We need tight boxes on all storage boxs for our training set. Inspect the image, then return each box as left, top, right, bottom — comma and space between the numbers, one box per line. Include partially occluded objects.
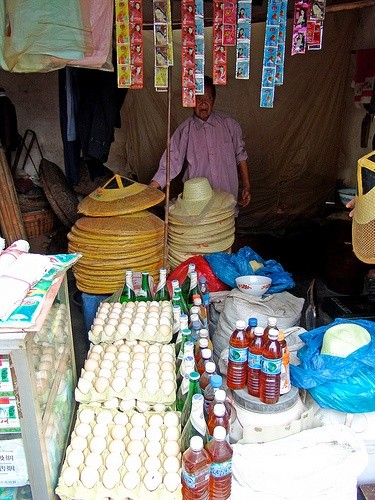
82, 293, 111, 347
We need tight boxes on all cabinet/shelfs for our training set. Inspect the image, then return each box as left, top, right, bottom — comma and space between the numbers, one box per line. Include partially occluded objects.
326, 212, 368, 296
0, 272, 78, 500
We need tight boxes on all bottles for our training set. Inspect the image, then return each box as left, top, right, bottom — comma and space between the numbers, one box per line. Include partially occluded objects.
227, 317, 291, 404
119, 263, 234, 500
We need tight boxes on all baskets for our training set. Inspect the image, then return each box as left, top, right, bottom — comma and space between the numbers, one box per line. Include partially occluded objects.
21, 209, 54, 238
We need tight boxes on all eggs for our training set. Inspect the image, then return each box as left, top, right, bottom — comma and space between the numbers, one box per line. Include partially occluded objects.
63, 301, 180, 499
33, 302, 69, 452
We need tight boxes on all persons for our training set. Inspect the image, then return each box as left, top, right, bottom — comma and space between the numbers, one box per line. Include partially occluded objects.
294, 8, 307, 52
150, 75, 252, 218
216, 2, 225, 83
117, 2, 130, 85
131, 2, 141, 84
0, 87, 23, 172
346, 150, 375, 218
184, 4, 195, 104
237, 7, 246, 76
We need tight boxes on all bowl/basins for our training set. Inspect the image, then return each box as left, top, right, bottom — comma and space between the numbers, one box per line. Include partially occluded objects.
338, 188, 359, 209
235, 275, 272, 298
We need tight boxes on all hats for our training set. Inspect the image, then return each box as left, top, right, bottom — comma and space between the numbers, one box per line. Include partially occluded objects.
310, 324, 375, 410
352, 150, 375, 264
67, 175, 235, 294
